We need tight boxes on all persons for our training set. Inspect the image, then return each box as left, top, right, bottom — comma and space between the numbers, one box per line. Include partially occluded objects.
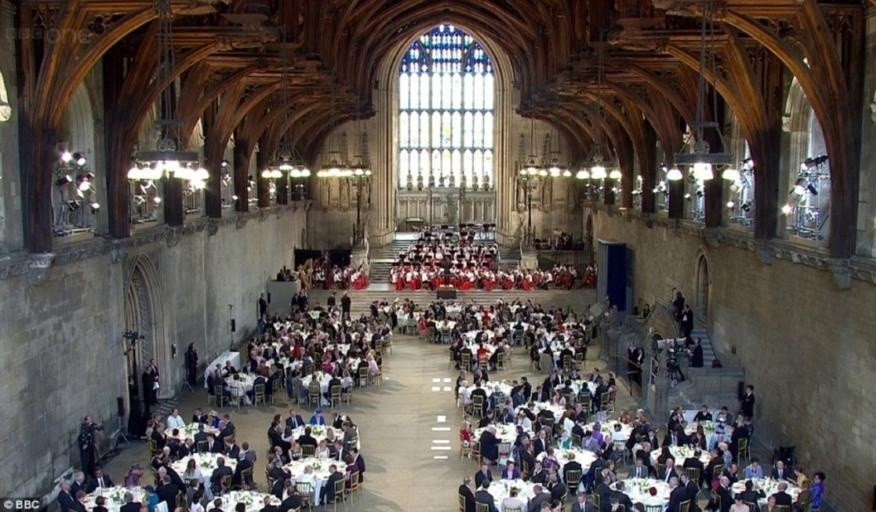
58, 234, 830, 511
277, 251, 370, 290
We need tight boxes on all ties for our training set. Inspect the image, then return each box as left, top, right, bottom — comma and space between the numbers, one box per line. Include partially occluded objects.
101, 478, 103, 488
